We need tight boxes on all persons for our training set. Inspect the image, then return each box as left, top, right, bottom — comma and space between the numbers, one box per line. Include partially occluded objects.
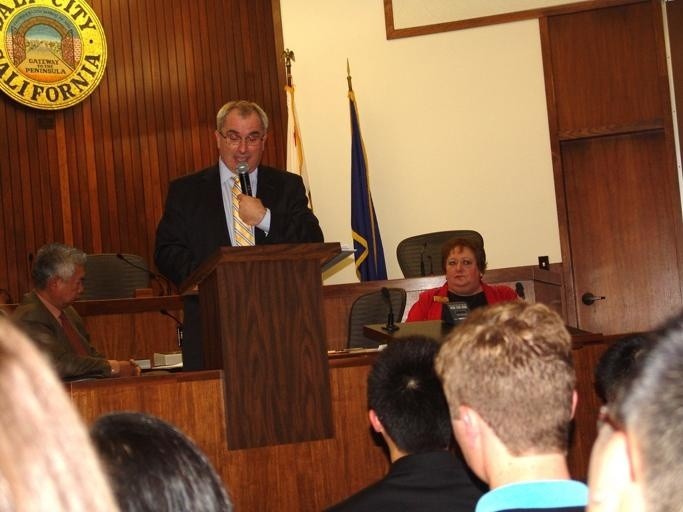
589, 312, 682, 512
323, 334, 488, 512
11, 243, 142, 381
0, 313, 121, 510
155, 100, 325, 369
434, 301, 588, 512
594, 333, 651, 405
406, 238, 520, 339
87, 410, 232, 510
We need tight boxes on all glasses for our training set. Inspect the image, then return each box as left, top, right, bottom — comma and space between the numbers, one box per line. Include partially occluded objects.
218, 131, 264, 145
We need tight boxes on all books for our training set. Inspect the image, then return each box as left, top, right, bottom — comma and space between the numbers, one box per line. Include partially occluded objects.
134, 351, 183, 370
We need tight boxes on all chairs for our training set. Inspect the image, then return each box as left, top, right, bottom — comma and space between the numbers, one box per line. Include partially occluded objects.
391, 230, 488, 277
342, 288, 406, 350
74, 253, 153, 298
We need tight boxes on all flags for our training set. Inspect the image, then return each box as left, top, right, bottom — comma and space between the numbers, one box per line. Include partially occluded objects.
285, 84, 313, 211
351, 89, 388, 281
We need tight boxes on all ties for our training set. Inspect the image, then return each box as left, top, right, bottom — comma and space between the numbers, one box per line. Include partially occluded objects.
232, 175, 253, 247
59, 311, 88, 357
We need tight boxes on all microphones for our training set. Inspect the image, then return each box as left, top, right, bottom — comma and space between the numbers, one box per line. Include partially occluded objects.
427, 256, 434, 276
420, 241, 427, 275
237, 163, 253, 196
160, 307, 182, 328
116, 252, 169, 284
382, 286, 398, 333
